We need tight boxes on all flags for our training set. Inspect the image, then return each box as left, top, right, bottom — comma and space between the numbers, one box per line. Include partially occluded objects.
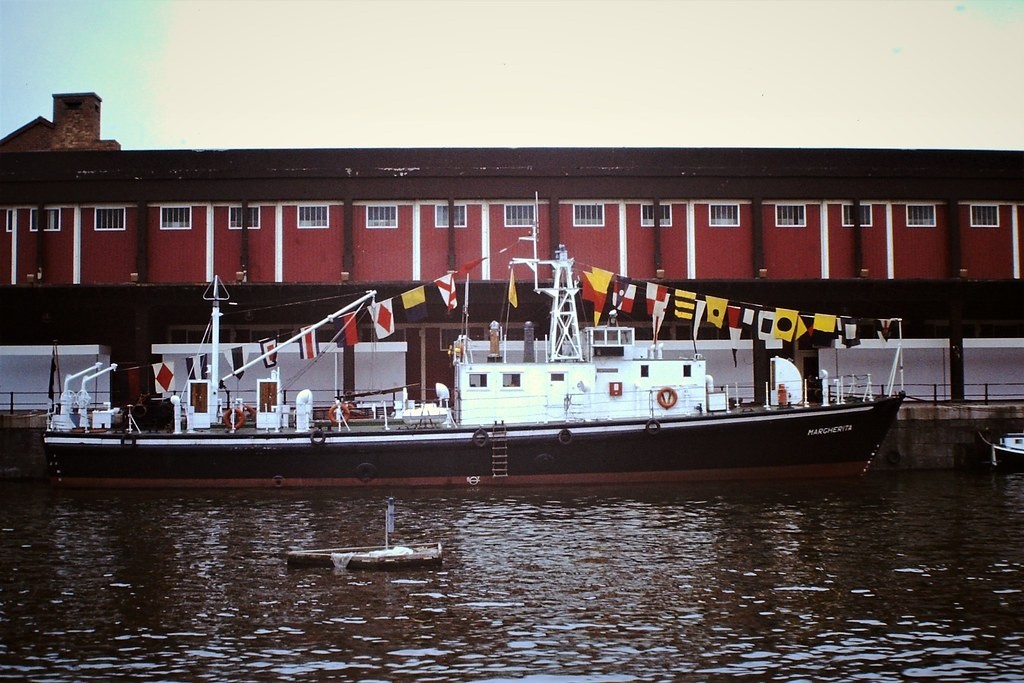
754, 306, 813, 350
675, 289, 696, 320
258, 335, 278, 369
185, 353, 208, 380
840, 316, 861, 346
812, 312, 836, 348
48, 347, 57, 409
298, 324, 320, 360
582, 270, 610, 305
737, 301, 763, 331
366, 297, 395, 340
646, 281, 669, 315
222, 343, 250, 381
333, 309, 359, 348
433, 273, 458, 309
705, 295, 729, 329
151, 360, 176, 394
401, 285, 426, 309
509, 267, 519, 309
612, 279, 637, 314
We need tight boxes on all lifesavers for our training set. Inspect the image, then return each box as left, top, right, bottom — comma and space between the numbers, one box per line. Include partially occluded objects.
355, 463, 377, 482
658, 387, 678, 407
557, 428, 573, 445
132, 404, 147, 418
329, 404, 351, 425
310, 429, 326, 445
646, 419, 661, 434
223, 408, 246, 429
473, 429, 489, 446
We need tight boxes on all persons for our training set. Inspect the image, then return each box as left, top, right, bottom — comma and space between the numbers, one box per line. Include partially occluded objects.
607, 309, 619, 327
334, 396, 341, 405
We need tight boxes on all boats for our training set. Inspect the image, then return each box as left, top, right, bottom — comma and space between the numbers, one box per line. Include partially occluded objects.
972, 428, 1024, 471
41, 189, 909, 489
